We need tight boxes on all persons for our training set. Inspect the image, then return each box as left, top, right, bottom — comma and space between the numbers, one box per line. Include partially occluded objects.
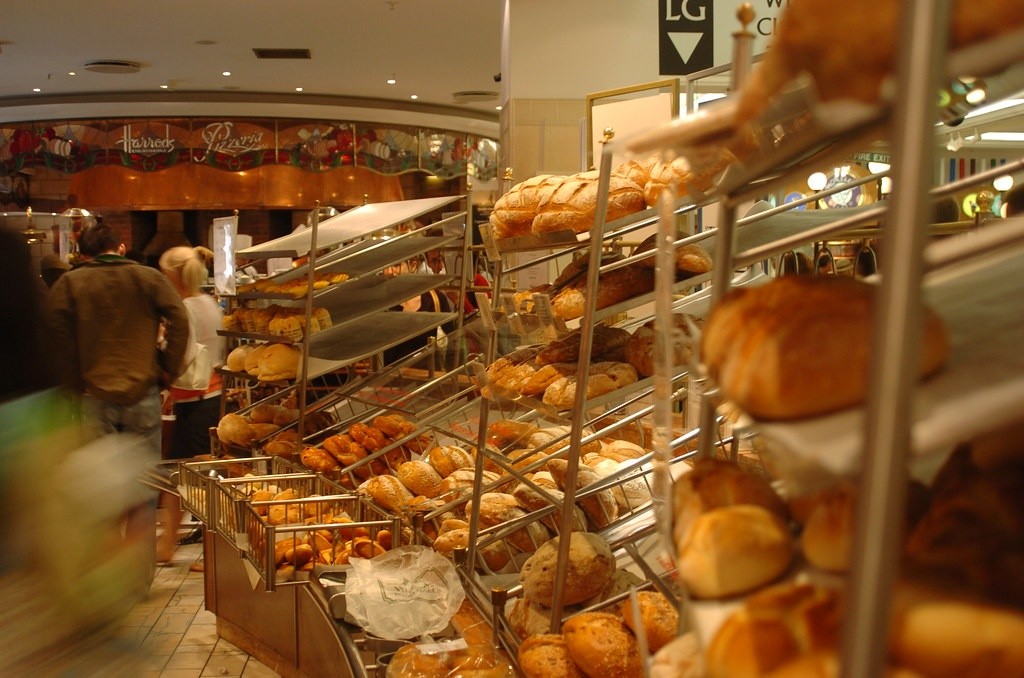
49, 224, 224, 571
384, 219, 493, 367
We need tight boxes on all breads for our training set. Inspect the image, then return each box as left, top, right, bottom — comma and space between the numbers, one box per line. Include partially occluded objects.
216, 271, 340, 458
190, 410, 439, 584
390, 0, 1024, 678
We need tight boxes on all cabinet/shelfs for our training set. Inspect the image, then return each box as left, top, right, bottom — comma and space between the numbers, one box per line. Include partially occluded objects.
142, 1, 1024, 677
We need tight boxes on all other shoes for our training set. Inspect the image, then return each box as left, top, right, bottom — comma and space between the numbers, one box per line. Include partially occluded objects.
155, 559, 173, 568
177, 528, 203, 545
